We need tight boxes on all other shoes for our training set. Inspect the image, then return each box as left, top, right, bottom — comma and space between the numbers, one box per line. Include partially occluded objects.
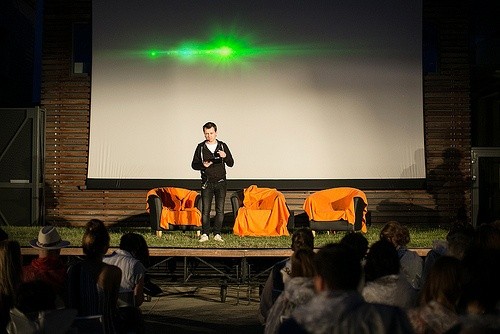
198, 234, 208, 244
213, 234, 224, 242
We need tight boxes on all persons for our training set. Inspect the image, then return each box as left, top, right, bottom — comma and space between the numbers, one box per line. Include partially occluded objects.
258, 228, 314, 323
103, 233, 150, 334
338, 220, 500, 333
263, 246, 316, 334
192, 122, 234, 243
0, 225, 76, 333
274, 242, 413, 334
60, 219, 122, 334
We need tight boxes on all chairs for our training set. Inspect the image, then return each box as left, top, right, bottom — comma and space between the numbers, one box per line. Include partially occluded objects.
230, 185, 295, 236
118, 290, 139, 333
302, 186, 369, 234
146, 187, 204, 236
74, 314, 105, 334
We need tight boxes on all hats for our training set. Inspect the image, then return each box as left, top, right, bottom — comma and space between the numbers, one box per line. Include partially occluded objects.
29, 227, 70, 250
